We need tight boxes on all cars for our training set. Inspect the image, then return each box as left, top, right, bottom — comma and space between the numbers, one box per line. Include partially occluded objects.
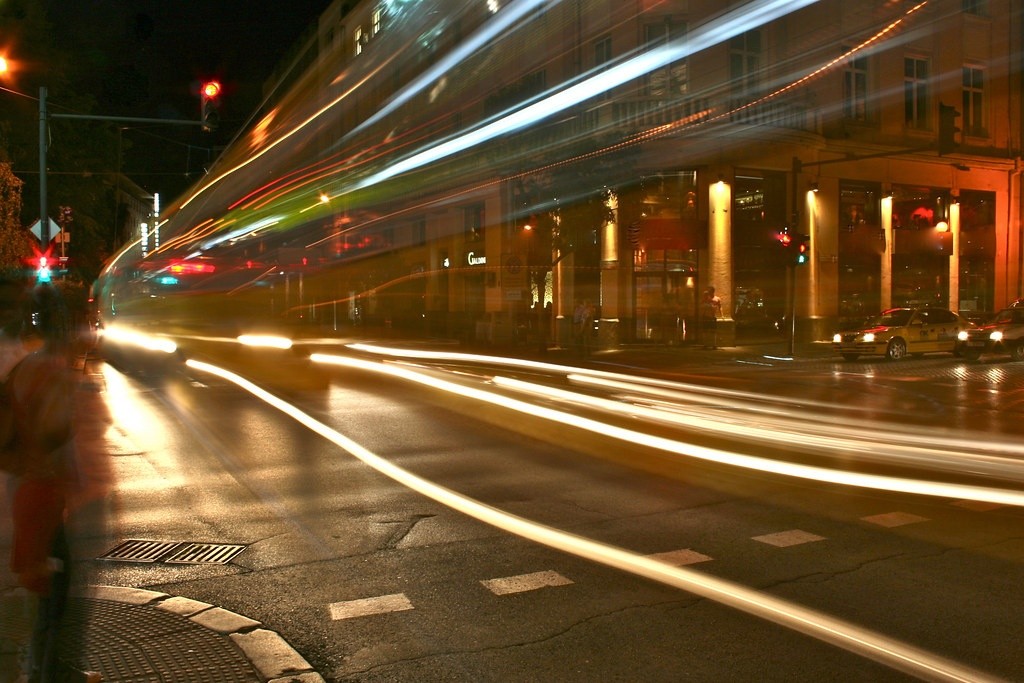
831, 303, 978, 361
955, 298, 1024, 361
733, 291, 978, 330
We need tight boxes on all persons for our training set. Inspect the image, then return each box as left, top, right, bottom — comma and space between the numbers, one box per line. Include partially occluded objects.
700, 291, 717, 319
573, 298, 590, 353
0, 287, 115, 683
702, 285, 725, 349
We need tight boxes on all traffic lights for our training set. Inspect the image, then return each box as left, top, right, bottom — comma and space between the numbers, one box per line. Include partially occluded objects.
202, 78, 220, 133
932, 217, 951, 258
936, 104, 960, 157
792, 233, 811, 267
34, 253, 55, 289
780, 222, 790, 250
